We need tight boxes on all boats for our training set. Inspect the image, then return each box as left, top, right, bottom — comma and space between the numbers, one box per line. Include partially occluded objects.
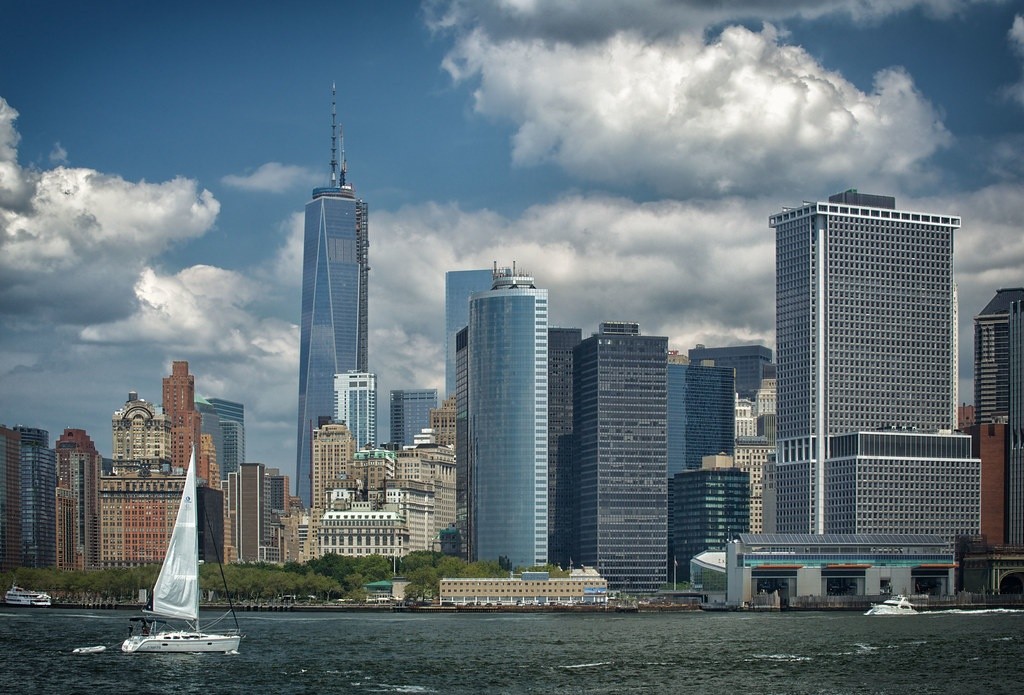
72, 644, 107, 655
863, 592, 920, 616
5, 586, 53, 608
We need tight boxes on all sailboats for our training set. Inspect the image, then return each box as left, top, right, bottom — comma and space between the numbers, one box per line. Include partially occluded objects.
117, 440, 242, 654
